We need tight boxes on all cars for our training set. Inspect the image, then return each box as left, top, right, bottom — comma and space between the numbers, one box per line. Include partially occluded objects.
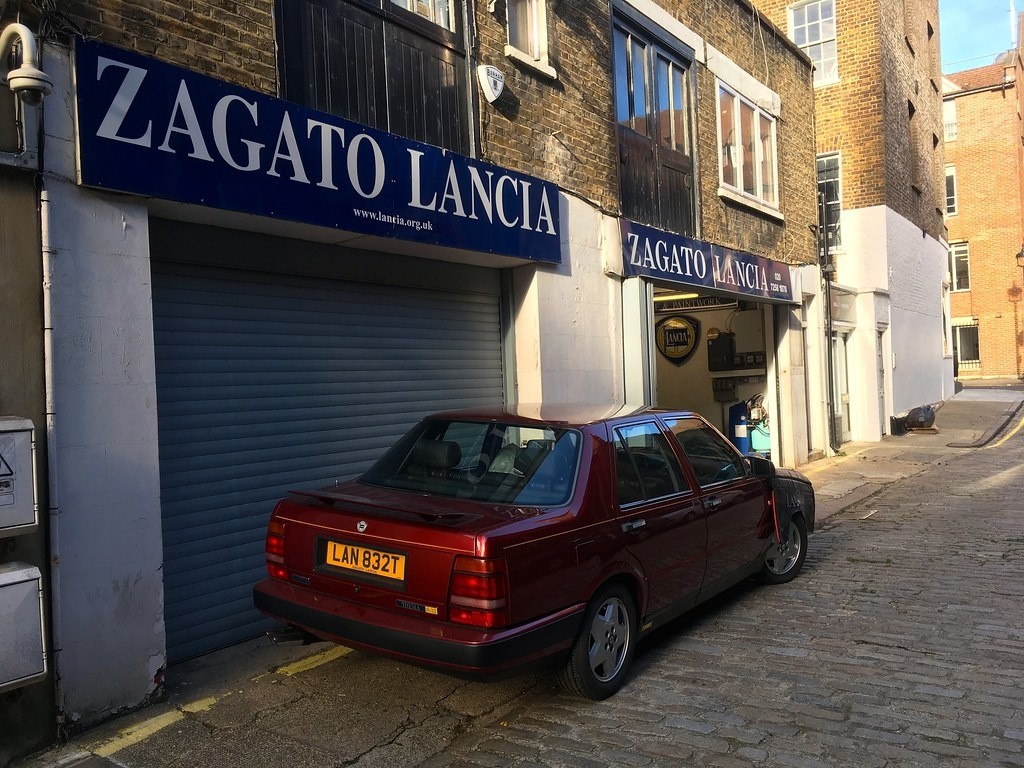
254, 400, 816, 704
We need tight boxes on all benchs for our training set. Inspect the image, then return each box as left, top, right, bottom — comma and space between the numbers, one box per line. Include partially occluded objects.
411, 440, 567, 504
